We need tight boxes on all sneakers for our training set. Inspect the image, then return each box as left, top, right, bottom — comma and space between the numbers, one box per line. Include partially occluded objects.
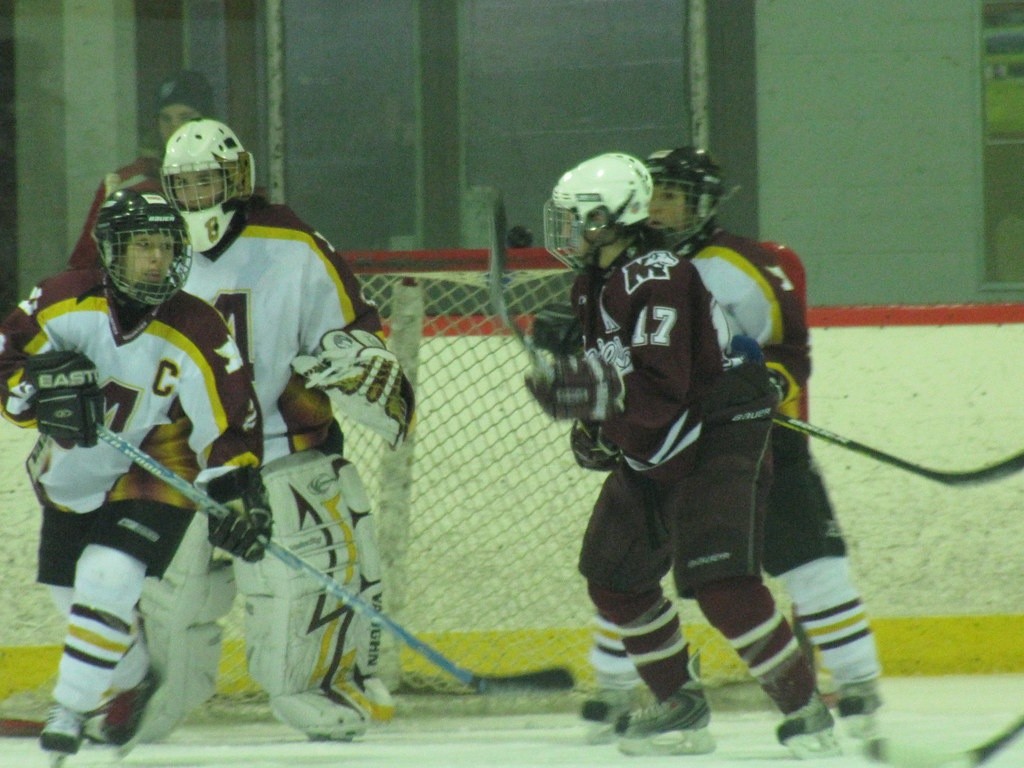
838, 680, 885, 753
97, 644, 176, 760
582, 673, 651, 745
777, 689, 842, 759
616, 650, 718, 755
41, 703, 88, 768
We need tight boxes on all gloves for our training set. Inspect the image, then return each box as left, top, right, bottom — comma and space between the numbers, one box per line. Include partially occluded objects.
525, 349, 625, 418
570, 420, 627, 470
531, 304, 575, 350
207, 465, 275, 566
769, 368, 788, 403
28, 349, 103, 448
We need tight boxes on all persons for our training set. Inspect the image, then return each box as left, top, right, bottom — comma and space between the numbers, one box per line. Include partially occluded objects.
0, 186, 274, 754
70, 72, 417, 741
521, 152, 839, 760
578, 147, 886, 759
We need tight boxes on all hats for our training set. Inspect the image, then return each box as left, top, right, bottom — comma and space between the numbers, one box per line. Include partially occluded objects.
158, 70, 213, 115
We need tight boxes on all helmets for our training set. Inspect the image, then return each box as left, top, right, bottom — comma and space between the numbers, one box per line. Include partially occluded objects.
646, 145, 729, 251
541, 153, 653, 271
162, 118, 255, 252
91, 187, 188, 304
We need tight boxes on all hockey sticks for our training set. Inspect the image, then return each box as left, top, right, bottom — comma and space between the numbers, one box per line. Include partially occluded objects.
864, 715, 1024, 767
94, 420, 578, 696
485, 192, 544, 370
765, 407, 1020, 489
